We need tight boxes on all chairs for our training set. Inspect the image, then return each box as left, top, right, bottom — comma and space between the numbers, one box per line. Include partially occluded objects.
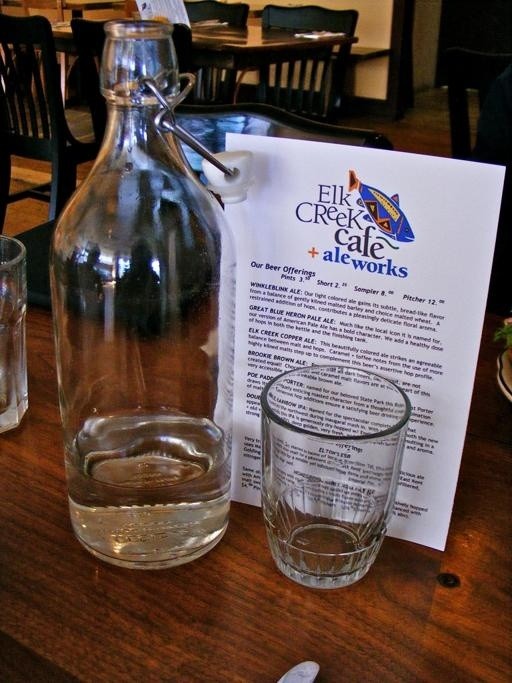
0, 13, 105, 222
260, 4, 359, 121
184, 0, 251, 106
442, 46, 510, 231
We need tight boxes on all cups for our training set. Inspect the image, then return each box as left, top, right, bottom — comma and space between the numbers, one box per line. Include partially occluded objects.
260, 362, 412, 592
0, 235, 30, 437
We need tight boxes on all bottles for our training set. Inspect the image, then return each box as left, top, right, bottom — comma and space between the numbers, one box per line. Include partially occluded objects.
48, 21, 238, 575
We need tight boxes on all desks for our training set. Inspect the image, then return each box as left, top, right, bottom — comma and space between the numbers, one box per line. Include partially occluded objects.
0, 238, 511, 683
0, 1, 361, 124
